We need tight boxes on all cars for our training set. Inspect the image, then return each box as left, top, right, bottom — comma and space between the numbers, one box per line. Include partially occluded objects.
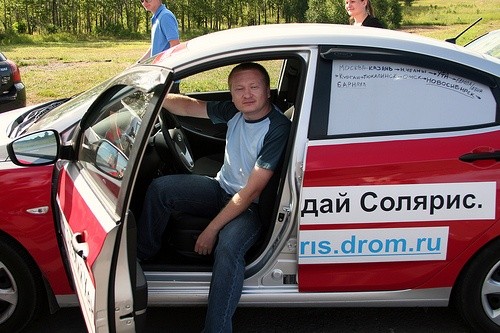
0, 23, 499, 333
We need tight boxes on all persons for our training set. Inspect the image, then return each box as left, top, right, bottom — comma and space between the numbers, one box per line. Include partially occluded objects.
345, 0, 384, 28
138, 1, 180, 94
137, 62, 292, 333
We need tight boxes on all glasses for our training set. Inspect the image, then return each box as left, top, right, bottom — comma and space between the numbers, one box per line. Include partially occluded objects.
140, 0, 151, 3
